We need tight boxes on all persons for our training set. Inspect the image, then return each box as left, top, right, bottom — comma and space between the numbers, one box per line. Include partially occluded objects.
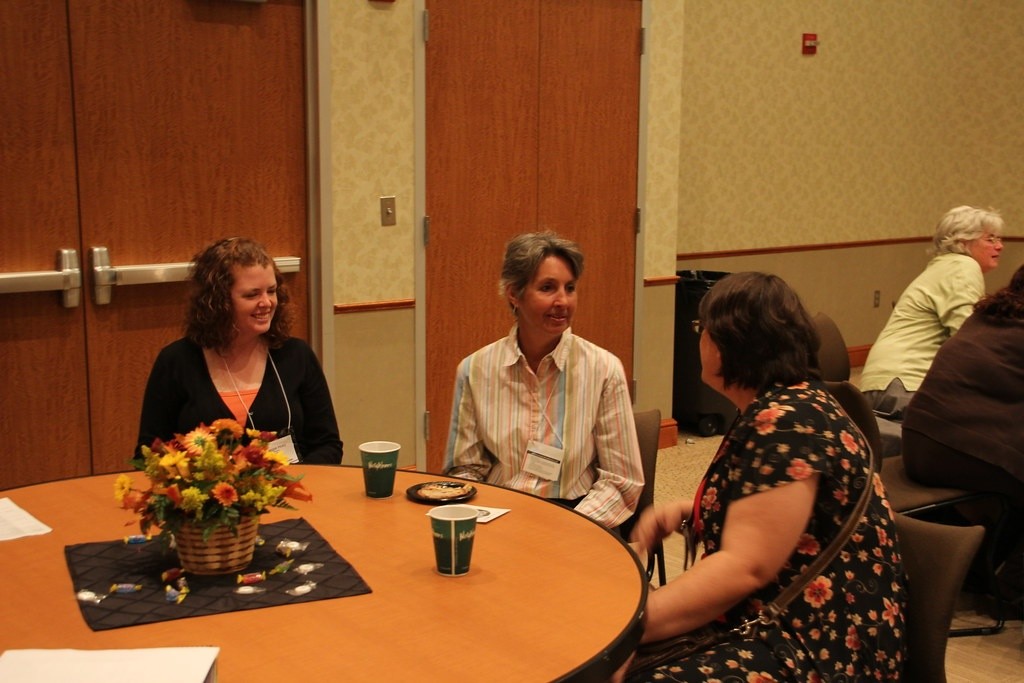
610, 270, 911, 683
131, 233, 343, 467
899, 262, 1024, 623
857, 206, 1005, 426
441, 229, 644, 540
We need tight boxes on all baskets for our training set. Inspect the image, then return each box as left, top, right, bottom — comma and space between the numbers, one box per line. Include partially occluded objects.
170, 514, 258, 575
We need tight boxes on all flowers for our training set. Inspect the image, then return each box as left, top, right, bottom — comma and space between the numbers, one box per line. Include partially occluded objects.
115, 418, 314, 556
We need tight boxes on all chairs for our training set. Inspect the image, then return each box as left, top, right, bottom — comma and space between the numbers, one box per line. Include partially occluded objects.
812, 310, 1009, 636
614, 411, 666, 587
892, 510, 986, 683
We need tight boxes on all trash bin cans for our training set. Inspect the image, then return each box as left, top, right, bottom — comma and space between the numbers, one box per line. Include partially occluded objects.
672, 270, 740, 436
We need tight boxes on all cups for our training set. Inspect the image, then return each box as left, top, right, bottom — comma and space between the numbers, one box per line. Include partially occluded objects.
358, 440, 401, 499
425, 504, 479, 577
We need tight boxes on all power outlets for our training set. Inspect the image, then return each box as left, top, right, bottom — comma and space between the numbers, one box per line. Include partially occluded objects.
874, 290, 880, 307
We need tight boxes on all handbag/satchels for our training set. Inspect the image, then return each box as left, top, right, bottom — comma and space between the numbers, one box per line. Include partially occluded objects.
623, 624, 717, 677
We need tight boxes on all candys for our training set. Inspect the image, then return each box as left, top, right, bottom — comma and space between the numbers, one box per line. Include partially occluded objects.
76, 535, 316, 604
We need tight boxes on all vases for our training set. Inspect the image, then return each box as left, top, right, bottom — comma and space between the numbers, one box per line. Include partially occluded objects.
173, 516, 259, 575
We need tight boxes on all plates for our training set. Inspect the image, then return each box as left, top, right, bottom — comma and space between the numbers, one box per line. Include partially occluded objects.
406, 481, 477, 504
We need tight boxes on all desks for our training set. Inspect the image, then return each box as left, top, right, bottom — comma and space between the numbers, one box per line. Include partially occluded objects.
0, 463, 649, 683
